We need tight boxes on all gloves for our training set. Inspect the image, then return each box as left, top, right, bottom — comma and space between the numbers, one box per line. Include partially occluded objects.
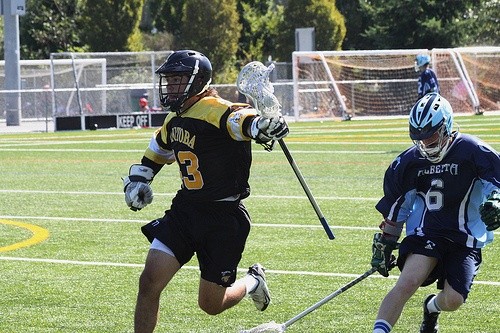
479, 193, 500, 232
370, 231, 398, 277
251, 115, 289, 144
122, 164, 156, 211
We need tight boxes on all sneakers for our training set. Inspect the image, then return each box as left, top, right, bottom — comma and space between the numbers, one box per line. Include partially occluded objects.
248, 262, 270, 312
420, 293, 441, 333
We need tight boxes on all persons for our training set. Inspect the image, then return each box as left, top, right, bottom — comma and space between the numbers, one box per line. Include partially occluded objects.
370, 91, 500, 333
139, 93, 150, 111
121, 49, 289, 333
414, 54, 440, 99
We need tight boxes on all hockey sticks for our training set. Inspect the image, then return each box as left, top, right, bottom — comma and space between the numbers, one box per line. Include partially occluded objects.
240, 255, 395, 333
235, 60, 335, 240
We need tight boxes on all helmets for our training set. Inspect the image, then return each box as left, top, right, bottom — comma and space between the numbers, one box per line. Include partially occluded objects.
155, 49, 213, 112
415, 54, 429, 71
408, 92, 453, 162
